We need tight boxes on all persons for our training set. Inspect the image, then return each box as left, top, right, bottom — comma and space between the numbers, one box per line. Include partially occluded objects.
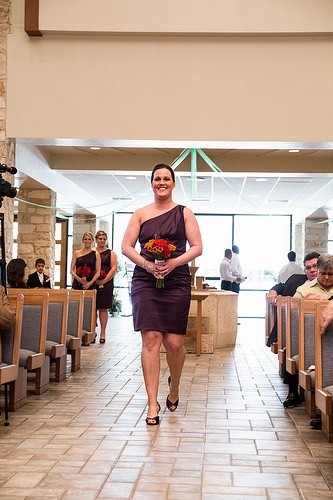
27, 258, 51, 289
70, 232, 101, 346
0, 285, 16, 327
278, 251, 304, 283
293, 254, 333, 427
320, 295, 333, 334
121, 164, 203, 424
7, 258, 28, 289
90, 231, 117, 343
220, 245, 242, 325
266, 252, 321, 407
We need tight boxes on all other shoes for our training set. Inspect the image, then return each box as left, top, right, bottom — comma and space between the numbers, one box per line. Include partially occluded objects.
310, 418, 323, 427
282, 390, 301, 407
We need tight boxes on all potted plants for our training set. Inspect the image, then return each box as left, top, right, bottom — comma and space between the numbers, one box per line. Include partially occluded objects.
108, 289, 122, 317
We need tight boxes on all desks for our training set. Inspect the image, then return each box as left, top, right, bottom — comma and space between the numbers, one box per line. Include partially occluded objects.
185, 295, 209, 358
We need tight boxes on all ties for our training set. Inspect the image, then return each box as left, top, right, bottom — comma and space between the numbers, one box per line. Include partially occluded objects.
38, 273, 44, 283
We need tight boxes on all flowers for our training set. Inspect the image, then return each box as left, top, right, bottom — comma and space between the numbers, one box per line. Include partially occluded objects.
144, 240, 176, 288
94, 270, 107, 288
76, 266, 91, 282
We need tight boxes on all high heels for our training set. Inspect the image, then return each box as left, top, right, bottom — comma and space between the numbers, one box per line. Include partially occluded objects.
145, 401, 161, 425
165, 376, 180, 412
99, 334, 106, 344
91, 334, 97, 344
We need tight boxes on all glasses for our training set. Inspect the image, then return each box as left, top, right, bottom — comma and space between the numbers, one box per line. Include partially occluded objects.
319, 271, 333, 276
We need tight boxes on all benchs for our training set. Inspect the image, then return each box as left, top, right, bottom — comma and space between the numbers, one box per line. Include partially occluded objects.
265, 293, 333, 443
0, 288, 97, 412
